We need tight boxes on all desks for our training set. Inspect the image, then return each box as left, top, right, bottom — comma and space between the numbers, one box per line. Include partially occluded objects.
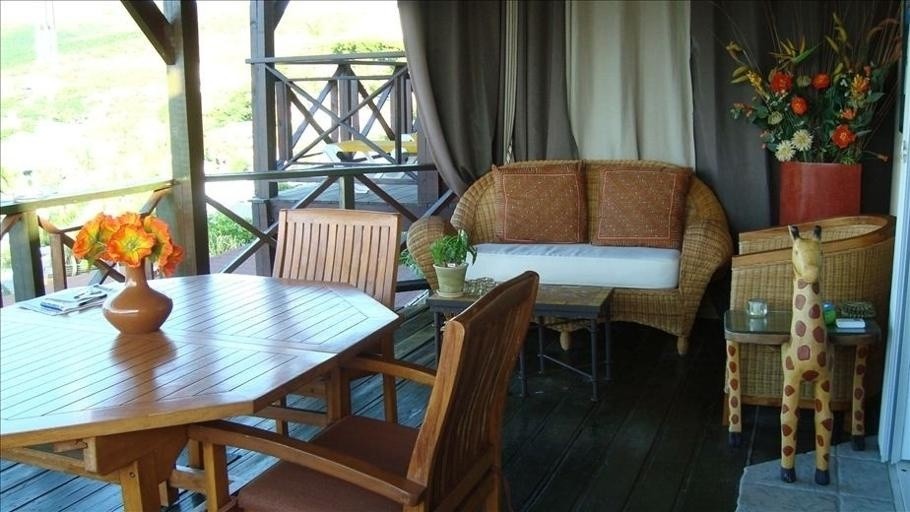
721, 224, 880, 450
3, 270, 402, 512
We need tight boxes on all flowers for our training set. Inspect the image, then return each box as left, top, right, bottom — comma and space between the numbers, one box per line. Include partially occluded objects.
725, 0, 910, 161
69, 210, 187, 277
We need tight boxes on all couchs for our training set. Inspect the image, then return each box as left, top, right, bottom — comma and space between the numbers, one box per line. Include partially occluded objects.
407, 158, 734, 360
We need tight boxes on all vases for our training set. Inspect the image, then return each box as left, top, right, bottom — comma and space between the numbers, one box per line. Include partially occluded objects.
101, 259, 177, 338
779, 161, 861, 227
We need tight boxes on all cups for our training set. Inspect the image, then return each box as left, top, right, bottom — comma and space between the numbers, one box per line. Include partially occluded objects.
746, 297, 766, 317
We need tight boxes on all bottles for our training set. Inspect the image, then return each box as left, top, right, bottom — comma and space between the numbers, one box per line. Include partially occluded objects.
822, 303, 836, 324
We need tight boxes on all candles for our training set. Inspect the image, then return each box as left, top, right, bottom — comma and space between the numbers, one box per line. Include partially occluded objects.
428, 227, 480, 298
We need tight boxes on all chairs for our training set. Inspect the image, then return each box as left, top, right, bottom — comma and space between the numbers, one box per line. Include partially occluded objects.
185, 268, 538, 512
325, 133, 419, 193
255, 207, 404, 443
722, 214, 897, 426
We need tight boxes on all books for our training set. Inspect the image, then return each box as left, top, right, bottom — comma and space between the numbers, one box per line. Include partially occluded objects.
20, 283, 113, 316
